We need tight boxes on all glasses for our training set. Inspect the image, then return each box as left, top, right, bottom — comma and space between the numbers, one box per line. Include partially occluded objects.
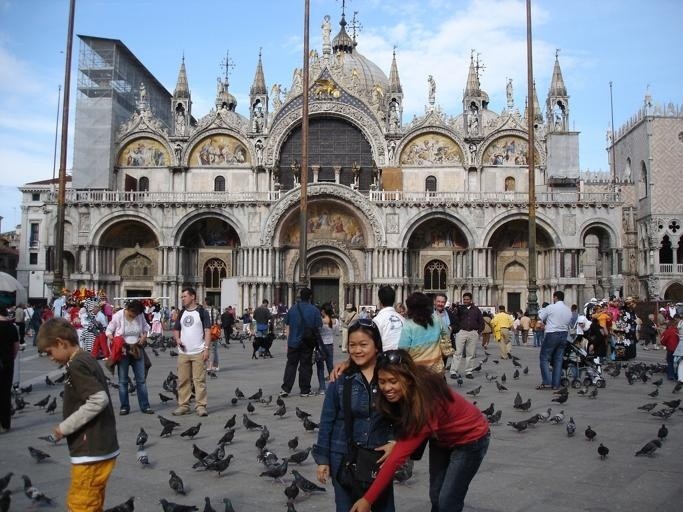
376, 351, 407, 365
348, 319, 377, 335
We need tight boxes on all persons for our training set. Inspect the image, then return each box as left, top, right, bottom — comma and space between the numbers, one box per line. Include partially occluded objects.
1, 287, 212, 435
205, 298, 683, 389
328, 350, 490, 512
279, 287, 323, 398
216, 78, 224, 98
505, 79, 513, 102
35, 318, 120, 511
146, 144, 166, 168
198, 137, 245, 167
387, 107, 398, 134
537, 290, 572, 389
125, 142, 146, 167
375, 286, 485, 379
320, 15, 332, 53
427, 75, 436, 97
139, 82, 146, 102
311, 318, 406, 511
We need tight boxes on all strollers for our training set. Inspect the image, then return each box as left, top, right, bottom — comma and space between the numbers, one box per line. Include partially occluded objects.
560, 334, 605, 390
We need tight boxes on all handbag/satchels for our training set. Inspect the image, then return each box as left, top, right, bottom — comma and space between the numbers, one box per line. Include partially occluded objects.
439, 335, 455, 357
337, 448, 385, 494
302, 328, 317, 346
125, 344, 142, 361
315, 343, 327, 365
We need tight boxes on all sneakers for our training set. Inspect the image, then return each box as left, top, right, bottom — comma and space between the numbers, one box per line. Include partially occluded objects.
173, 404, 191, 416
196, 409, 209, 417
144, 408, 155, 415
302, 390, 317, 397
119, 408, 129, 416
279, 389, 289, 397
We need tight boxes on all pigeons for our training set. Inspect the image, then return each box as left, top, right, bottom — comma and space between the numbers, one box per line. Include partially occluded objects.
598, 443, 609, 460
218, 332, 255, 349
37, 431, 61, 445
466, 350, 683, 441
145, 332, 179, 358
0, 490, 12, 512
21, 474, 52, 508
394, 466, 413, 485
9, 372, 66, 418
106, 372, 326, 512
28, 446, 51, 463
0, 472, 14, 494
635, 440, 662, 458
457, 378, 463, 387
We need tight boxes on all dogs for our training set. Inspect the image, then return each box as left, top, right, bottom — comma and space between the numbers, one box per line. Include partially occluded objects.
250, 333, 277, 359
